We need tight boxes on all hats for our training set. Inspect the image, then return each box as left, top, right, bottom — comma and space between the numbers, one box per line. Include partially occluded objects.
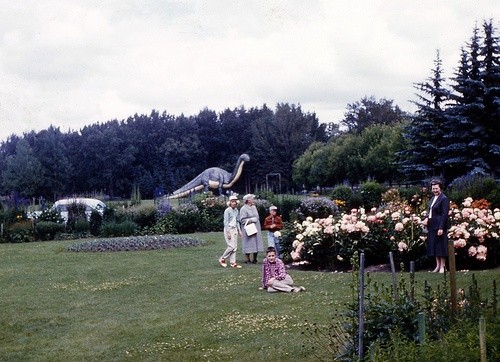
243, 193, 256, 200
227, 196, 240, 206
269, 206, 278, 210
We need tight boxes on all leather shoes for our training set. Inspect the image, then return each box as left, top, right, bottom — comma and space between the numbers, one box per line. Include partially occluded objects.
245, 259, 258, 265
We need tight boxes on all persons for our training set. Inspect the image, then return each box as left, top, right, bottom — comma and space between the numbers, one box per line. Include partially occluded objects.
240, 194, 264, 264
264, 205, 284, 258
424, 179, 449, 273
219, 196, 244, 269
258, 247, 305, 292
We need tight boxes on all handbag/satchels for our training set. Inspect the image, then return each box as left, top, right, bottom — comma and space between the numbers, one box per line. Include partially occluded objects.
243, 219, 258, 237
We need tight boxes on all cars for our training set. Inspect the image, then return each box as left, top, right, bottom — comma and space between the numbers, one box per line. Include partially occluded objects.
27, 198, 107, 225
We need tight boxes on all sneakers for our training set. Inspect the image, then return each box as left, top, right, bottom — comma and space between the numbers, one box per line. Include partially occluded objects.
292, 286, 306, 292
230, 262, 241, 269
218, 258, 226, 268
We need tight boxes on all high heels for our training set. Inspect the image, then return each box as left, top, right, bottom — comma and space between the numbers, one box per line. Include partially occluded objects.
433, 265, 445, 274
